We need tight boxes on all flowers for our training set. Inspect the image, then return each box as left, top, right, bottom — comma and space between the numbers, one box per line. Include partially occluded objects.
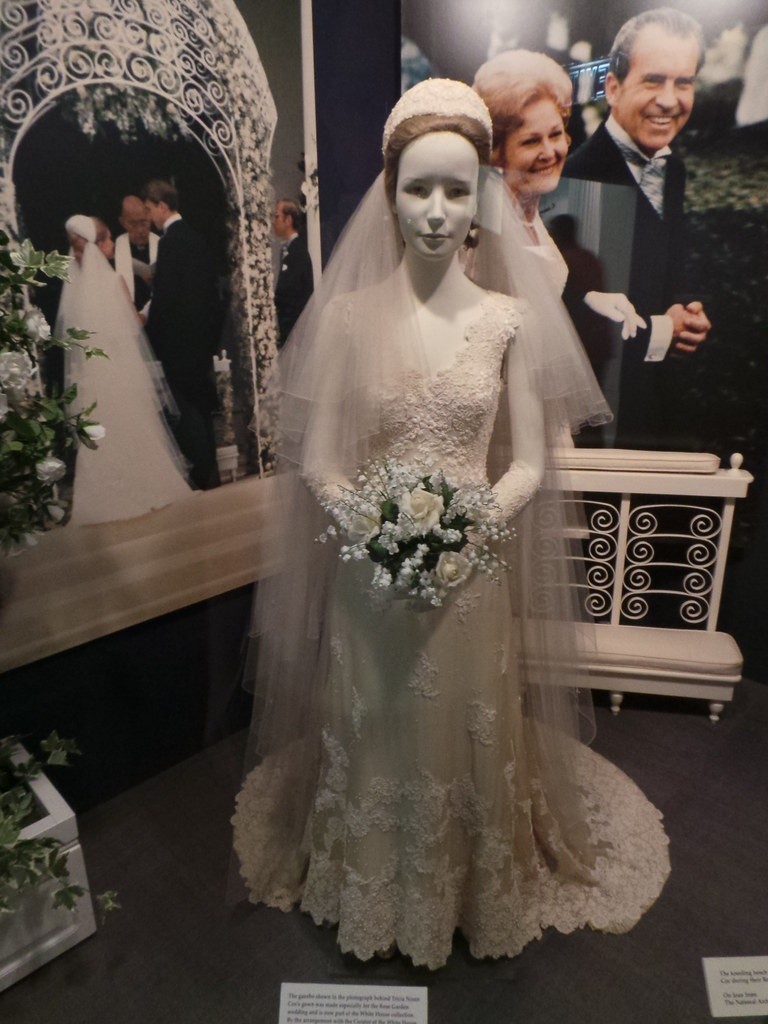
316, 457, 515, 618
0, 235, 107, 534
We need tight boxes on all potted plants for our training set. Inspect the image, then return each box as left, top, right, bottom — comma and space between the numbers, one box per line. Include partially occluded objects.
0, 739, 98, 991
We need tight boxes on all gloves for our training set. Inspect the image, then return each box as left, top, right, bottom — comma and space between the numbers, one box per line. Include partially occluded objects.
584, 288, 647, 340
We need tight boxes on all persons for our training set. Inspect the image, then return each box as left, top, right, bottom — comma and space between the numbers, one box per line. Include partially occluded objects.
141, 180, 219, 491
272, 200, 314, 344
233, 78, 668, 967
468, 49, 648, 341
107, 195, 159, 326
540, 9, 711, 448
58, 215, 195, 528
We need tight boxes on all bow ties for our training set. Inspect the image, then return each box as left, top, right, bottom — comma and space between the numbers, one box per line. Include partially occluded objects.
614, 137, 666, 184
